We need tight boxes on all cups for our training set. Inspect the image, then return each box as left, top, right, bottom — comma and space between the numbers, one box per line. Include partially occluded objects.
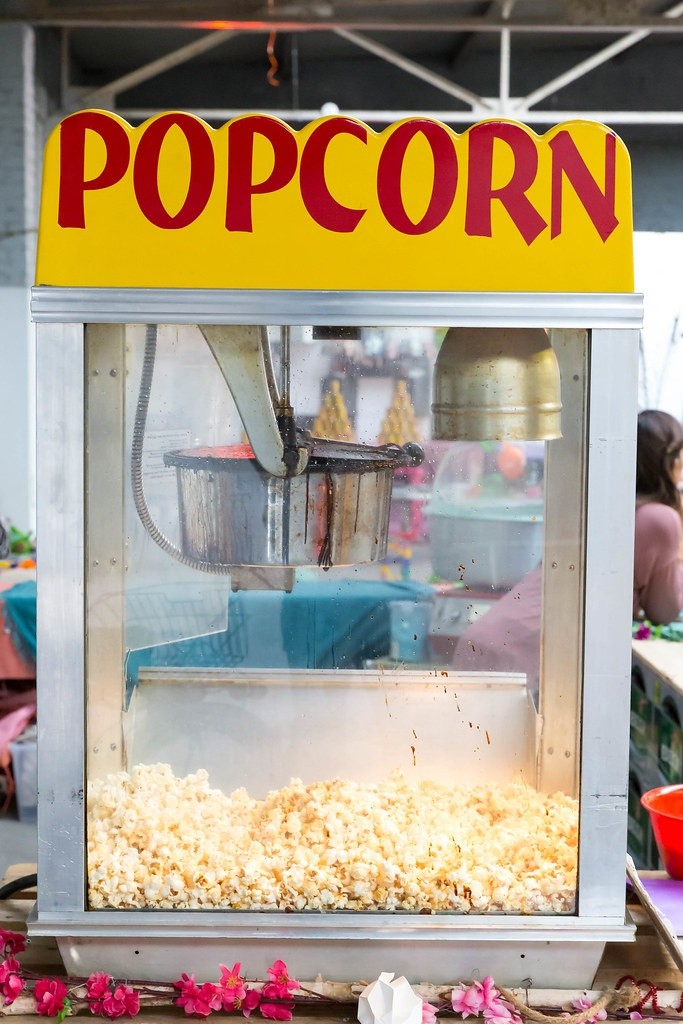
388, 600, 429, 662
6, 741, 38, 823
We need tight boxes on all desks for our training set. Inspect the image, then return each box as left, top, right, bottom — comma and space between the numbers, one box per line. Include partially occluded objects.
0, 568, 437, 681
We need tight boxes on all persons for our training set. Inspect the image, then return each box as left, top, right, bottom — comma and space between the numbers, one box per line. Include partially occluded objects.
453, 411, 683, 711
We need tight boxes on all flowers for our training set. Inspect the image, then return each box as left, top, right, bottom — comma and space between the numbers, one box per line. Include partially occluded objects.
0, 928, 683, 1024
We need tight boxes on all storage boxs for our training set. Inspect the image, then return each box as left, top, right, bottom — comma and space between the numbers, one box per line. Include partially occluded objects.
420, 440, 546, 589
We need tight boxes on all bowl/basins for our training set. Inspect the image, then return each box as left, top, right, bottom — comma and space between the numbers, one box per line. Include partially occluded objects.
421, 506, 544, 588
640, 784, 683, 879
